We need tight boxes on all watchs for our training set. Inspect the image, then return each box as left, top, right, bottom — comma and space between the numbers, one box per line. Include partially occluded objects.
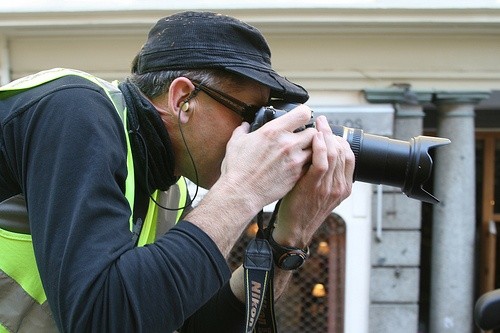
260, 228, 311, 271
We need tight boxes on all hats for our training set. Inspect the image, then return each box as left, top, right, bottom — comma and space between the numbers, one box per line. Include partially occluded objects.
131, 10, 309, 106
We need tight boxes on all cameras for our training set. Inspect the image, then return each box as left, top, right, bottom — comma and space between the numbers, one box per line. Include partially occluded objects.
248, 98, 451, 206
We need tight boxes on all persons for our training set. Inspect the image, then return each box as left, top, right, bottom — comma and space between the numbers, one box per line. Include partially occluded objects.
0, 11, 357, 333
